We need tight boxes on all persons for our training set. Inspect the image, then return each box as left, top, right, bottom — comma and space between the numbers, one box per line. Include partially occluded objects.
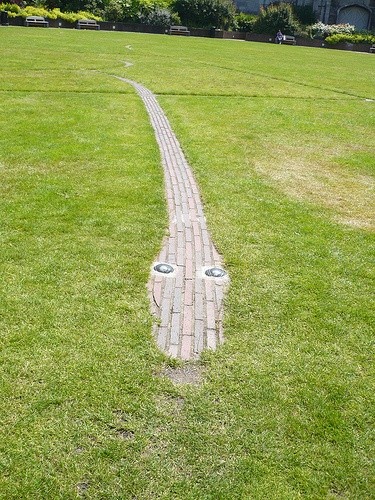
277, 29, 284, 45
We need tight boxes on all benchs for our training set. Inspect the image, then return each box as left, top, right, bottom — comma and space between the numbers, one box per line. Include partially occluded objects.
275, 35, 296, 45
77, 19, 100, 30
369, 44, 375, 53
23, 16, 49, 28
169, 26, 190, 36
